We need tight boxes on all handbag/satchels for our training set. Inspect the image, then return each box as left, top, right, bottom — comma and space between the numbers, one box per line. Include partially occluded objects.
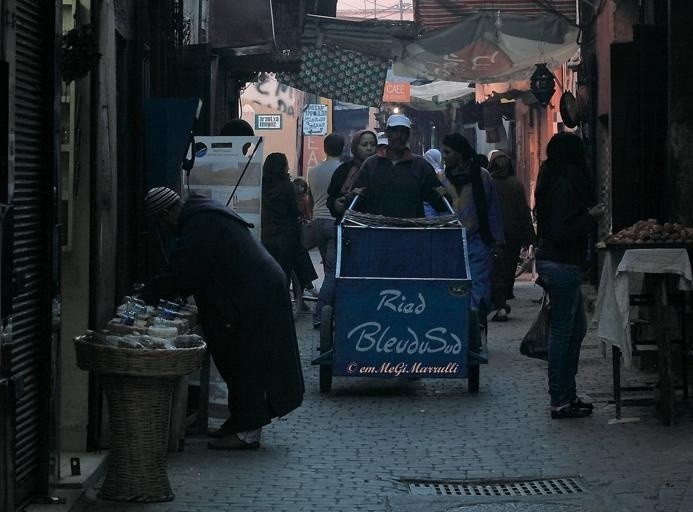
519, 291, 552, 361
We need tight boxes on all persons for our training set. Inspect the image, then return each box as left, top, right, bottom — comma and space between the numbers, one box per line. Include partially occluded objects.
136, 186, 306, 451
533, 134, 607, 416
261, 113, 533, 362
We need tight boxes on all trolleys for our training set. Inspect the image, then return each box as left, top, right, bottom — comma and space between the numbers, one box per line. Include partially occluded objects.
312, 187, 487, 393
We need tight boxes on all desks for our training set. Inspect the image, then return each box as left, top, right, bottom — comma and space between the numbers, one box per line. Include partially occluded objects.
85, 328, 210, 448
606, 248, 693, 426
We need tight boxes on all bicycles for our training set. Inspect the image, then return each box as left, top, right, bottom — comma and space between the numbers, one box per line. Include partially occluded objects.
287, 265, 306, 324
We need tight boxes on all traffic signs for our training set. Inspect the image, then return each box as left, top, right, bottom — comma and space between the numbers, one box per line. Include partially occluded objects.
304, 105, 327, 135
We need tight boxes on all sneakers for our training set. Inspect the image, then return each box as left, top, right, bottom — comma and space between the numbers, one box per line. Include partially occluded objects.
301, 287, 318, 300
295, 301, 317, 314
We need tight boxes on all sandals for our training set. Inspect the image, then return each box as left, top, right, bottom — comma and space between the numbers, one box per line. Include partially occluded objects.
206, 424, 235, 439
491, 306, 507, 322
205, 432, 261, 449
550, 406, 592, 419
570, 398, 593, 410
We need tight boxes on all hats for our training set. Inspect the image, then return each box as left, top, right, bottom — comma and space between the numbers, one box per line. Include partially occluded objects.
386, 114, 411, 129
141, 186, 181, 219
376, 132, 388, 145
